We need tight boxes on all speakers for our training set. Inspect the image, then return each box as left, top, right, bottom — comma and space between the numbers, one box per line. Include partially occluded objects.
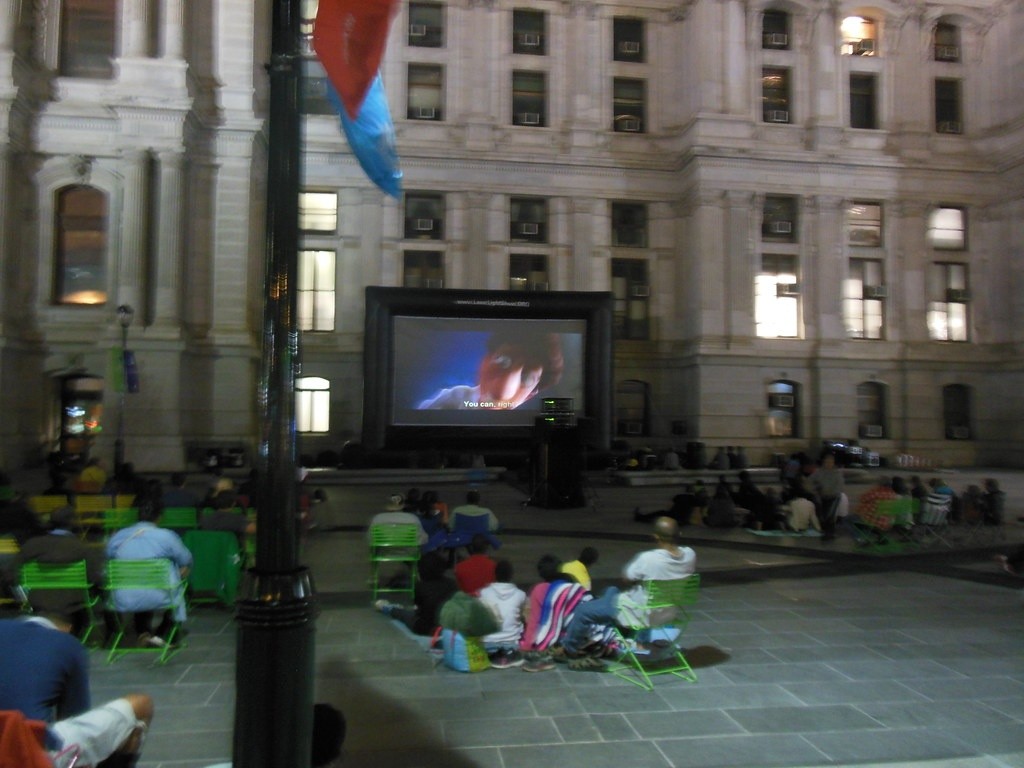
532, 429, 587, 509
672, 420, 688, 435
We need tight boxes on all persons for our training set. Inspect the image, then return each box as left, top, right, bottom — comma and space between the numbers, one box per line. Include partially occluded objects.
893, 475, 1007, 528
44, 456, 256, 552
204, 703, 346, 768
0, 694, 154, 768
0, 597, 91, 728
549, 516, 696, 657
366, 488, 598, 673
843, 476, 895, 545
635, 443, 849, 533
814, 454, 844, 541
12, 505, 99, 613
297, 488, 324, 525
107, 500, 193, 648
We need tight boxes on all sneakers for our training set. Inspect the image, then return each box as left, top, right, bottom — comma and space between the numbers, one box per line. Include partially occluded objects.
523, 641, 608, 672
488, 647, 524, 669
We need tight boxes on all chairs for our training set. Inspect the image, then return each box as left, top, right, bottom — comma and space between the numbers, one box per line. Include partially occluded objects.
848, 497, 954, 555
602, 570, 705, 690
0, 478, 261, 768
367, 512, 502, 605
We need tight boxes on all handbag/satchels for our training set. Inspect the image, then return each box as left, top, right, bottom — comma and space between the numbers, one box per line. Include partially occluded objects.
438, 590, 500, 638
431, 625, 491, 673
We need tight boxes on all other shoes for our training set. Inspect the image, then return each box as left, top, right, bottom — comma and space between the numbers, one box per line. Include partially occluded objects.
376, 598, 395, 611
136, 631, 151, 648
149, 636, 176, 648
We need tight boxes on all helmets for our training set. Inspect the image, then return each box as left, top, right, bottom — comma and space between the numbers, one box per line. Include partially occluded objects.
385, 491, 406, 511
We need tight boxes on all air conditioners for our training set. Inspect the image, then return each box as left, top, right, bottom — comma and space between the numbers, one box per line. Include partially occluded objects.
767, 111, 789, 123
765, 32, 789, 46
770, 221, 792, 233
522, 33, 540, 46
776, 283, 798, 296
865, 284, 888, 300
949, 287, 972, 302
410, 21, 445, 288
936, 46, 960, 62
940, 118, 960, 134
518, 113, 540, 125
860, 37, 874, 52
620, 40, 640, 52
532, 281, 549, 291
522, 223, 538, 235
619, 117, 640, 132
634, 283, 650, 297
620, 393, 970, 438
